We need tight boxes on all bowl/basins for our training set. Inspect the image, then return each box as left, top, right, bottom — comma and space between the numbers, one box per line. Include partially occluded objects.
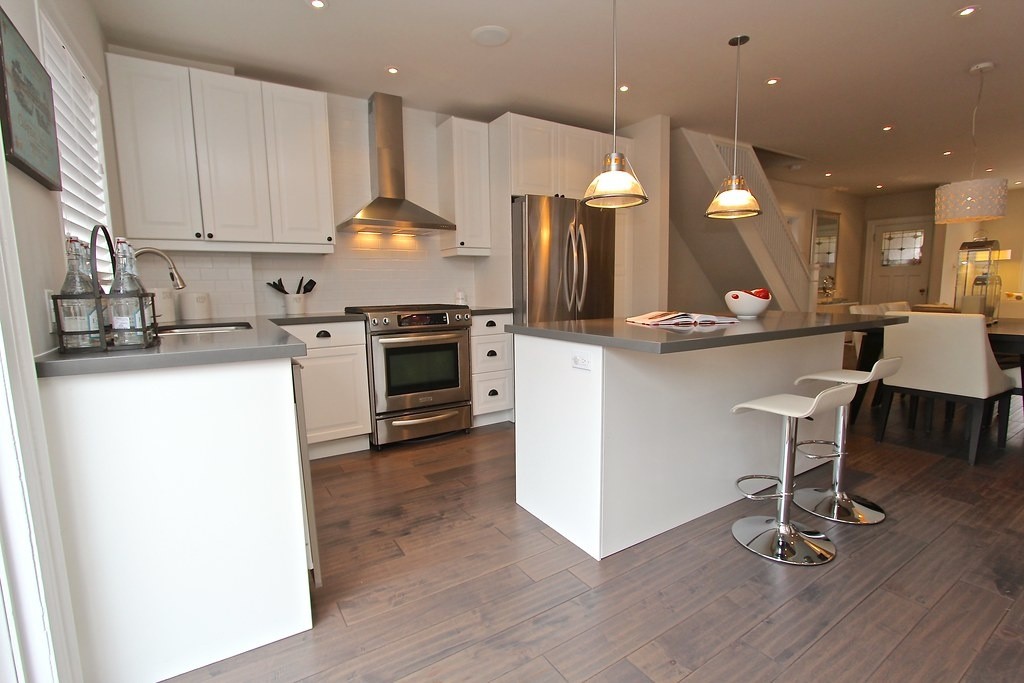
726, 290, 771, 319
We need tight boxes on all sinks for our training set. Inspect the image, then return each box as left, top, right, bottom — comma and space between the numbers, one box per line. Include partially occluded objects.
817, 300, 856, 305
158, 321, 254, 336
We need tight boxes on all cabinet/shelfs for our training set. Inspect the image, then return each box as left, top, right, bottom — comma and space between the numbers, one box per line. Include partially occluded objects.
38, 321, 371, 683
105, 52, 336, 254
470, 313, 515, 428
437, 110, 637, 257
816, 302, 860, 342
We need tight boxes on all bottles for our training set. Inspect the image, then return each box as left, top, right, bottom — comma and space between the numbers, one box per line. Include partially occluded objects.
60, 236, 153, 347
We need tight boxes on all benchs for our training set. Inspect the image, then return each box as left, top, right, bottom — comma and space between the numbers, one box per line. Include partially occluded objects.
848, 296, 1024, 408
875, 307, 1016, 467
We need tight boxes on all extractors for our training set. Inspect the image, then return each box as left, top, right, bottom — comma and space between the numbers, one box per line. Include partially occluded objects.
337, 93, 457, 236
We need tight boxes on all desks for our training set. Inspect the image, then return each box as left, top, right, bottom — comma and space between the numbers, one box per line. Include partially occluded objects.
847, 317, 1024, 425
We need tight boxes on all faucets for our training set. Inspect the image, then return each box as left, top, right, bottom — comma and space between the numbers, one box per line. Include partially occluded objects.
134, 247, 187, 290
823, 275, 837, 297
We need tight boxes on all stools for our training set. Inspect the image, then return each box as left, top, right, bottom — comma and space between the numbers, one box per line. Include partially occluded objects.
730, 355, 903, 566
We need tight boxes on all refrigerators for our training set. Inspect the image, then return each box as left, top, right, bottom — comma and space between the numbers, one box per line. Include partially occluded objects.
512, 193, 615, 324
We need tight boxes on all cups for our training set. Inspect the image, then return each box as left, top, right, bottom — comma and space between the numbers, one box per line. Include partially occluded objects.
179, 292, 211, 319
283, 294, 306, 315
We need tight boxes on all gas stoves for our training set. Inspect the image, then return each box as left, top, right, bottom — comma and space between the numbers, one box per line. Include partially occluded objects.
345, 303, 473, 334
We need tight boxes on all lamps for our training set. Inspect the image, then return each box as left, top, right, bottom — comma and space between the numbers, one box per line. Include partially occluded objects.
579, 0, 648, 210
703, 34, 763, 219
934, 62, 1008, 224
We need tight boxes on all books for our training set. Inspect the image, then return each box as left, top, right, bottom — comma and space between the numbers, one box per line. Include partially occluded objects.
625, 311, 741, 325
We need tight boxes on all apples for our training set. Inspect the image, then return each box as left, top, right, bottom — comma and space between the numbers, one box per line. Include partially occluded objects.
731, 289, 769, 299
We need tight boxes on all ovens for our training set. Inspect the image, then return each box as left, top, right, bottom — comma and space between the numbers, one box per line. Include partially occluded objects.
367, 329, 473, 450
